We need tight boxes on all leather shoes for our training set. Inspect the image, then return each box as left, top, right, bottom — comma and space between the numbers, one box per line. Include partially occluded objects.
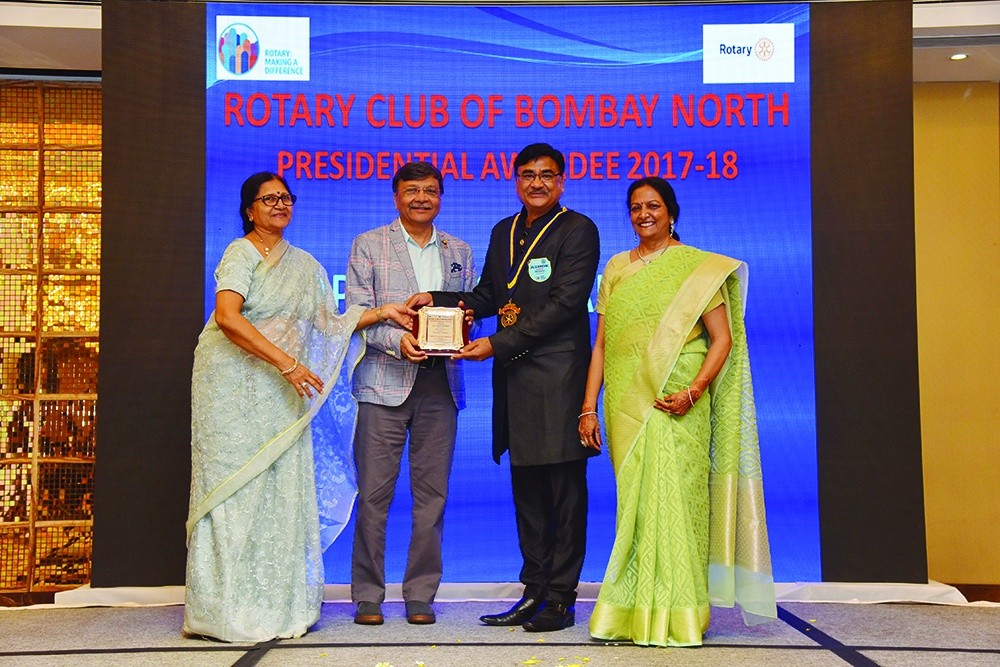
522, 603, 575, 632
479, 596, 541, 626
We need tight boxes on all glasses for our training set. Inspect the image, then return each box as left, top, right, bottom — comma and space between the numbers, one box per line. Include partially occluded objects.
516, 172, 561, 181
251, 193, 296, 206
396, 185, 437, 196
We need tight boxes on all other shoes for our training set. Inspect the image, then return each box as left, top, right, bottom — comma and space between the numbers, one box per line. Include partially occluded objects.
355, 601, 383, 623
405, 601, 436, 623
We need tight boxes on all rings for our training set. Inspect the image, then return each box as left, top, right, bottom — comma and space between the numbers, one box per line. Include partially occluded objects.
666, 410, 671, 416
302, 381, 307, 388
580, 439, 588, 448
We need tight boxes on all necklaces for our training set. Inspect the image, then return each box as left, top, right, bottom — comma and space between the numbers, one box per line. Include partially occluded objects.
636, 240, 670, 264
254, 230, 283, 255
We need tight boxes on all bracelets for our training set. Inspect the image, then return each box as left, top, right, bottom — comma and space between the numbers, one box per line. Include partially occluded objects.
687, 387, 695, 406
578, 411, 599, 417
376, 305, 387, 323
278, 357, 298, 376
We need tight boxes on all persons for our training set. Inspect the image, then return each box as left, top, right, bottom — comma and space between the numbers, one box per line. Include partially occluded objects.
345, 161, 479, 625
404, 143, 601, 632
578, 176, 777, 647
182, 171, 418, 644
